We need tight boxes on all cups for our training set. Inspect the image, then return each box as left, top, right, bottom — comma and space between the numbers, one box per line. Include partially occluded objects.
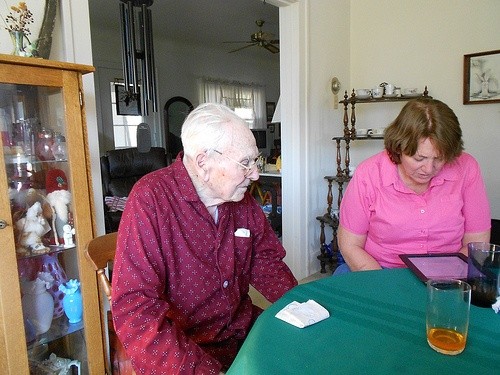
426, 279, 472, 356
463, 242, 500, 308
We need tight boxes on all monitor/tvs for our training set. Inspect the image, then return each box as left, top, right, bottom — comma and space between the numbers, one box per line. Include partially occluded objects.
250, 128, 272, 157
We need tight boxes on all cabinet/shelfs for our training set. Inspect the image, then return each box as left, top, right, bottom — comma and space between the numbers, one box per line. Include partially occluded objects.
315, 82, 433, 273
0, 55, 108, 374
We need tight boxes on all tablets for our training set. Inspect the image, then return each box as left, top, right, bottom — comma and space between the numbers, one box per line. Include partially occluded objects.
398, 252, 498, 289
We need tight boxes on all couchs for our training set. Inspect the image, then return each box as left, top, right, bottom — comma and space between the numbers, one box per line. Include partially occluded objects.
100, 147, 168, 234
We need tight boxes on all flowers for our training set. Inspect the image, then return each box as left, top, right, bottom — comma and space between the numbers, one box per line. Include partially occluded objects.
0, 0, 34, 45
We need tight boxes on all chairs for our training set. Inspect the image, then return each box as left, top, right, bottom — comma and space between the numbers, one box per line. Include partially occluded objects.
85, 232, 132, 375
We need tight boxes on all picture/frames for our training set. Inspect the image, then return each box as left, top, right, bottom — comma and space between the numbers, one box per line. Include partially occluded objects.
266, 102, 276, 121
463, 50, 500, 105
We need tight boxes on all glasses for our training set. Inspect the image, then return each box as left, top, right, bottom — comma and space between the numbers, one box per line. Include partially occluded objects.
204, 149, 264, 175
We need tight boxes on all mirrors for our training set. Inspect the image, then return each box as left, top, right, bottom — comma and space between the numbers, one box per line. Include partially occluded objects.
163, 96, 194, 165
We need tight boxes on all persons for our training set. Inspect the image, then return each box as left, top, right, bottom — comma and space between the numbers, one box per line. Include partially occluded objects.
111, 102, 299, 375
333, 99, 491, 276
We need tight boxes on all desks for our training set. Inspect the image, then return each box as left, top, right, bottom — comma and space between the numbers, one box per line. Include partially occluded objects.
251, 173, 283, 238
226, 268, 500, 375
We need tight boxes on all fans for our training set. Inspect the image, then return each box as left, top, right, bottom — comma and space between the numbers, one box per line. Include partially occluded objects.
219, 20, 280, 54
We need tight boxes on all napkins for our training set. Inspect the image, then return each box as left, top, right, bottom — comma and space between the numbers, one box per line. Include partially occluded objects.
275, 299, 330, 329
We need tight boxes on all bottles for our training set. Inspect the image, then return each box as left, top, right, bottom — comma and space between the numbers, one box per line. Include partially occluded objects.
51, 136, 68, 162
37, 251, 68, 319
21, 288, 55, 335
37, 130, 55, 161
62, 287, 83, 324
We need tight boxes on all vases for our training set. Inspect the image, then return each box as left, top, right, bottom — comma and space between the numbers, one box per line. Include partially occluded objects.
39, 251, 69, 320
19, 272, 55, 336
9, 32, 27, 55
58, 279, 83, 323
